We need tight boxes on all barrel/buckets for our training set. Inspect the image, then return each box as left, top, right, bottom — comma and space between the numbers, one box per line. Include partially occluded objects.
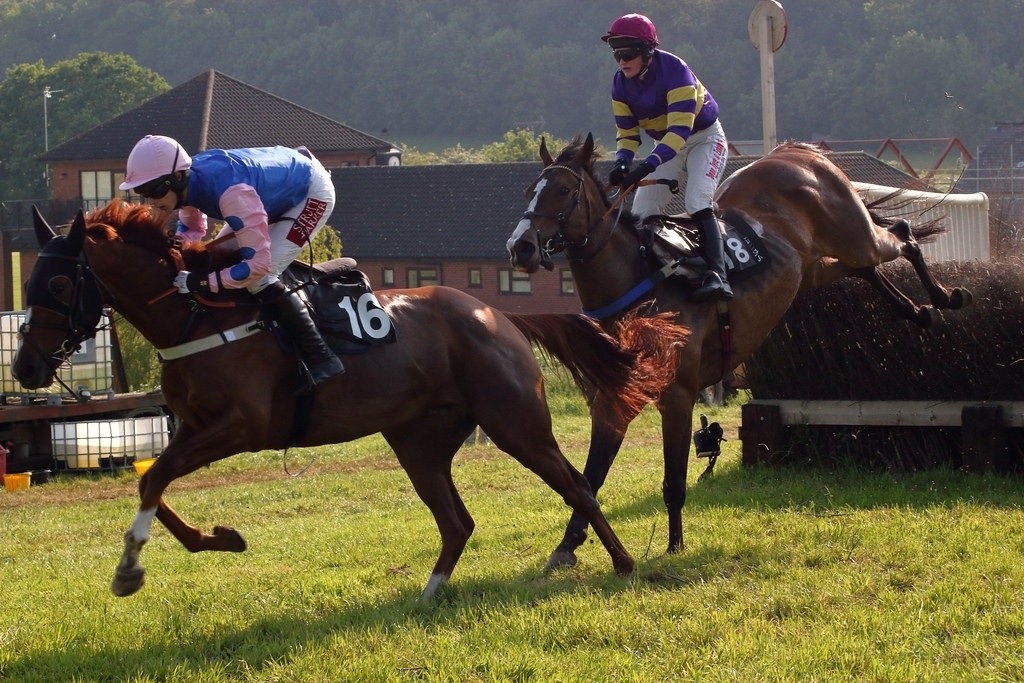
3, 472, 32, 495
133, 458, 157, 478
28, 470, 52, 486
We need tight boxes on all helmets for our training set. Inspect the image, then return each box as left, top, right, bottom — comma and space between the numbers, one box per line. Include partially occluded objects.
601, 13, 659, 47
118, 135, 192, 190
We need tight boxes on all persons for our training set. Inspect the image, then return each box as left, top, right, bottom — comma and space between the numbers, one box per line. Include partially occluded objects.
601, 14, 734, 304
118, 132, 345, 396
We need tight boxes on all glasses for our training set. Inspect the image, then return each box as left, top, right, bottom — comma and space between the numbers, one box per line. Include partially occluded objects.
141, 181, 169, 199
614, 47, 643, 63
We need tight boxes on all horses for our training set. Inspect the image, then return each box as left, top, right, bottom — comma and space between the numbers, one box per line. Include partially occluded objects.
11, 202, 637, 612
504, 133, 976, 580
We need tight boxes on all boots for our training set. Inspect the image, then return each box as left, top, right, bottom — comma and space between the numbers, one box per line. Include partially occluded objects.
279, 292, 346, 384
691, 209, 733, 300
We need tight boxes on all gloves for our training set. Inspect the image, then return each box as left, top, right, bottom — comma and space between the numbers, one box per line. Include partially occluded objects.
623, 162, 656, 195
172, 270, 219, 293
609, 159, 629, 185
